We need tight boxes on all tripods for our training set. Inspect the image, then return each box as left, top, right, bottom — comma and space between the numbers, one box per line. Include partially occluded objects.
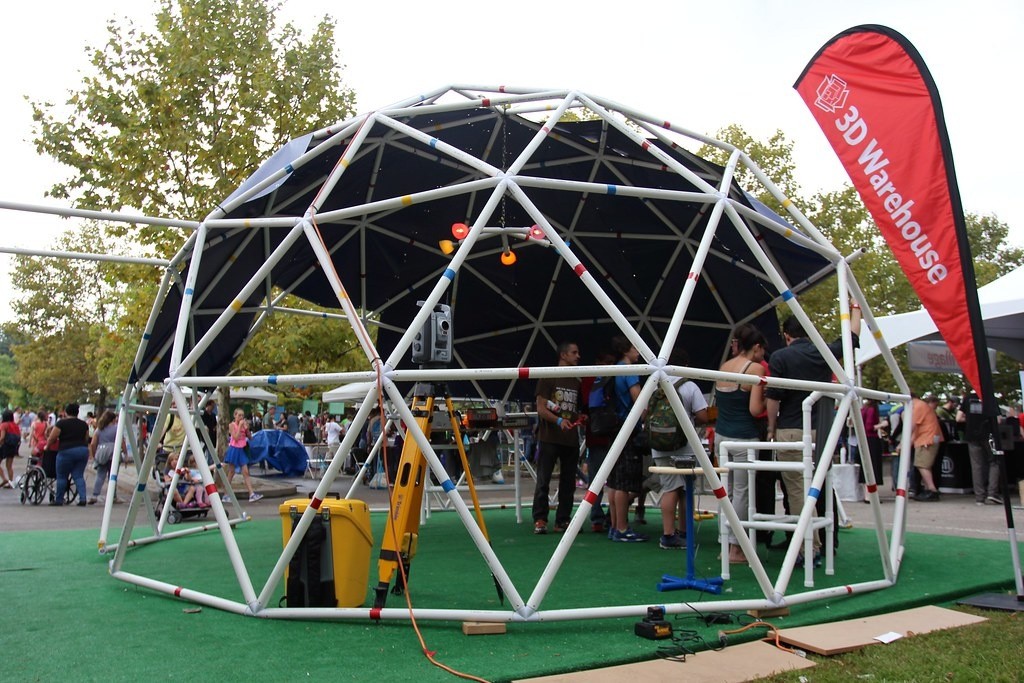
373, 363, 504, 610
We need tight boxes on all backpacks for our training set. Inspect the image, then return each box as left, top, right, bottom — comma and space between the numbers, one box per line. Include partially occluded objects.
644, 377, 689, 452
588, 376, 622, 437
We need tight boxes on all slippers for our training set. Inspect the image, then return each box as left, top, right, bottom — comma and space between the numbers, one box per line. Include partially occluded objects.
864, 499, 883, 504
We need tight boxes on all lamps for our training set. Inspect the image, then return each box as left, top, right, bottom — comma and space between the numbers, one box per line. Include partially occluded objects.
438, 105, 545, 265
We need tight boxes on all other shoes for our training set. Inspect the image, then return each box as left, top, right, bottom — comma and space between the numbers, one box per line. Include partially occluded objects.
0, 480, 12, 489
176, 502, 185, 509
729, 553, 750, 563
221, 495, 231, 502
114, 497, 126, 504
186, 500, 196, 508
249, 493, 264, 502
592, 514, 613, 532
533, 519, 584, 534
196, 501, 211, 508
913, 490, 940, 501
976, 494, 1004, 505
76, 501, 86, 506
88, 497, 98, 504
48, 501, 63, 506
794, 548, 823, 568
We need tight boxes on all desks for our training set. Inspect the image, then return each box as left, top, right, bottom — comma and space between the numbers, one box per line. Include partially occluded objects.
303, 442, 360, 481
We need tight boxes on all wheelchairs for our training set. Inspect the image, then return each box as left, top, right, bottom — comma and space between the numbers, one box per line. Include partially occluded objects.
16, 447, 79, 506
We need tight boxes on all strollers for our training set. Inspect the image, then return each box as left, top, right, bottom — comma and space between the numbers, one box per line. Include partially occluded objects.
151, 443, 231, 524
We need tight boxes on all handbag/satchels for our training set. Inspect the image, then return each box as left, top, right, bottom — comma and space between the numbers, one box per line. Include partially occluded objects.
369, 460, 388, 489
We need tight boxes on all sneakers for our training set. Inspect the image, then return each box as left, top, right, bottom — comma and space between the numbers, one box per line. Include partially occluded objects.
608, 526, 650, 542
659, 530, 687, 550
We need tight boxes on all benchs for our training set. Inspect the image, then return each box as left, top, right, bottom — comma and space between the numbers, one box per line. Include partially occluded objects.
306, 459, 332, 463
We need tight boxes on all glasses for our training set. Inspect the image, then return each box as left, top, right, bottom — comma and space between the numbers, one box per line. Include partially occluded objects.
731, 338, 739, 344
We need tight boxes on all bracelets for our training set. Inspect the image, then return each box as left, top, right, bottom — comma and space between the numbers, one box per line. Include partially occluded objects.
851, 304, 860, 309
557, 417, 562, 425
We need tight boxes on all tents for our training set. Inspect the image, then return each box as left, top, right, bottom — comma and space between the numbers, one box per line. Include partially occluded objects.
855, 263, 1024, 406
314, 379, 423, 480
120, 376, 278, 416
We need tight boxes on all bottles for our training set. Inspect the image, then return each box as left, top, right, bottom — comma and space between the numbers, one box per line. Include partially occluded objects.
546, 400, 562, 414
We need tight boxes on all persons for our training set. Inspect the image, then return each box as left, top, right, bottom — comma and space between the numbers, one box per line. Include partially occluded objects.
847, 385, 1024, 508
221, 407, 263, 503
522, 335, 718, 549
250, 405, 468, 482
156, 393, 226, 508
0, 404, 149, 506
714, 298, 861, 570
